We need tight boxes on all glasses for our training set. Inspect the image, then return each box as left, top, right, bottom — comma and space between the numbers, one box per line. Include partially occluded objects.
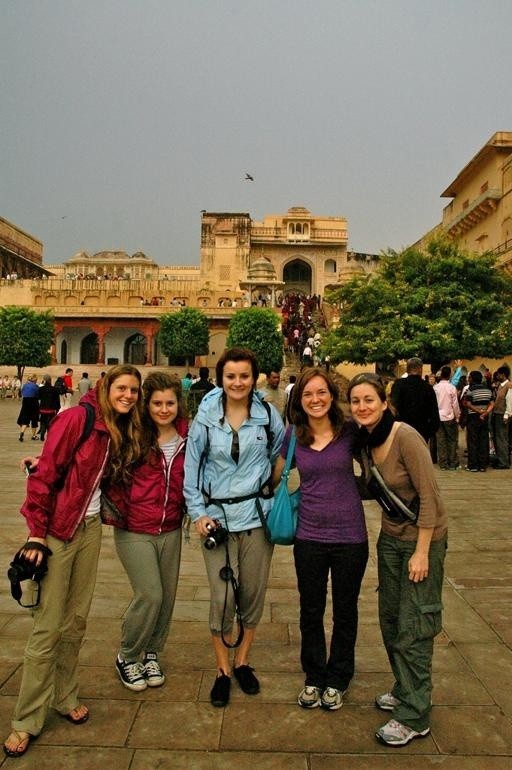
349, 373, 384, 385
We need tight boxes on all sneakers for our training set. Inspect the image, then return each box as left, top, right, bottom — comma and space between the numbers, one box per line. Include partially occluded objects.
375, 718, 430, 748
298, 686, 324, 708
320, 687, 347, 710
211, 668, 230, 706
142, 652, 164, 686
115, 653, 147, 692
233, 665, 259, 695
375, 692, 402, 712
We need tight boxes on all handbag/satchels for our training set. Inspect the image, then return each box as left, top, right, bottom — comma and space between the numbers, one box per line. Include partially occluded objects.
367, 476, 405, 521
265, 477, 302, 545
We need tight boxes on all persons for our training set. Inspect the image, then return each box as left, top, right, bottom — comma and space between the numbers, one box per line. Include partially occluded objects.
17, 372, 191, 692
183, 366, 216, 418
181, 347, 286, 708
73, 272, 125, 281
276, 293, 331, 373
17, 368, 105, 442
140, 296, 186, 307
347, 370, 451, 747
31, 272, 47, 281
0, 374, 21, 398
256, 367, 297, 417
262, 295, 267, 307
267, 368, 364, 712
389, 357, 512, 474
3, 362, 146, 758
6, 271, 17, 280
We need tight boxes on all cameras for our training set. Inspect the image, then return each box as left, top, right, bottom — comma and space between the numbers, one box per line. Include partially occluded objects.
7, 555, 49, 583
204, 520, 229, 550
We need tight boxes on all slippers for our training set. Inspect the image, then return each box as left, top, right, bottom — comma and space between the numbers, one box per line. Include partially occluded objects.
64, 704, 88, 724
3, 731, 31, 757
